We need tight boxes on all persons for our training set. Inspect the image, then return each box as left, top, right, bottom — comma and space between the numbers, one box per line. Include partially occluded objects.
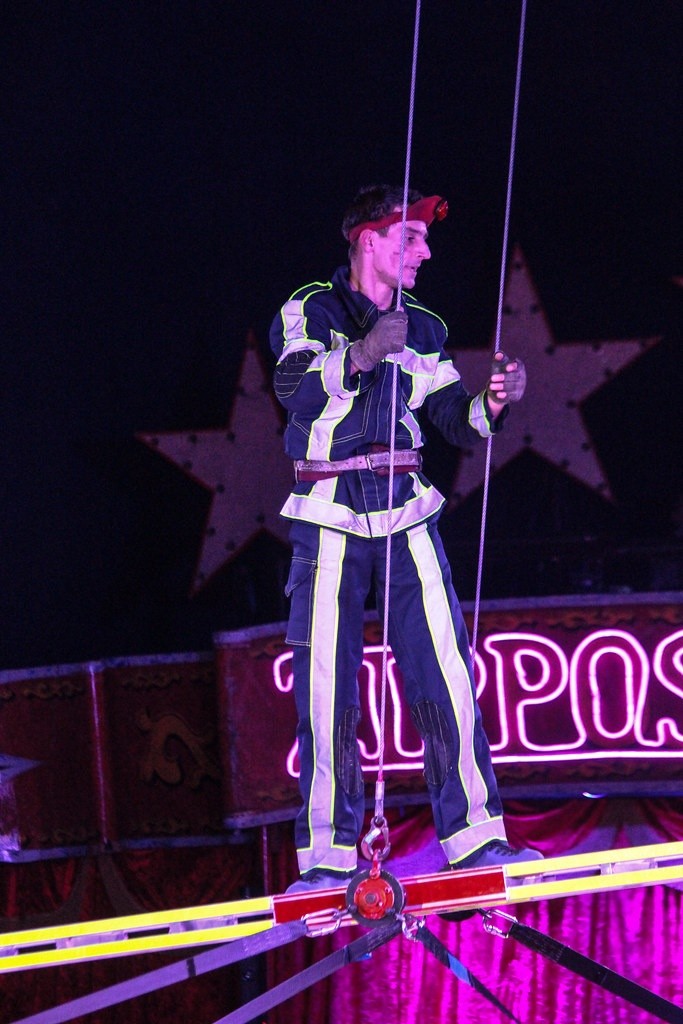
270, 184, 543, 897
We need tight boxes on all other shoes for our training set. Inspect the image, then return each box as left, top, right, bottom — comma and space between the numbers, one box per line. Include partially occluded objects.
460, 839, 544, 869
285, 867, 351, 896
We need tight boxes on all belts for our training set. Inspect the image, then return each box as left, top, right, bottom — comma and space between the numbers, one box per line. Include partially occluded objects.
293, 451, 420, 472
